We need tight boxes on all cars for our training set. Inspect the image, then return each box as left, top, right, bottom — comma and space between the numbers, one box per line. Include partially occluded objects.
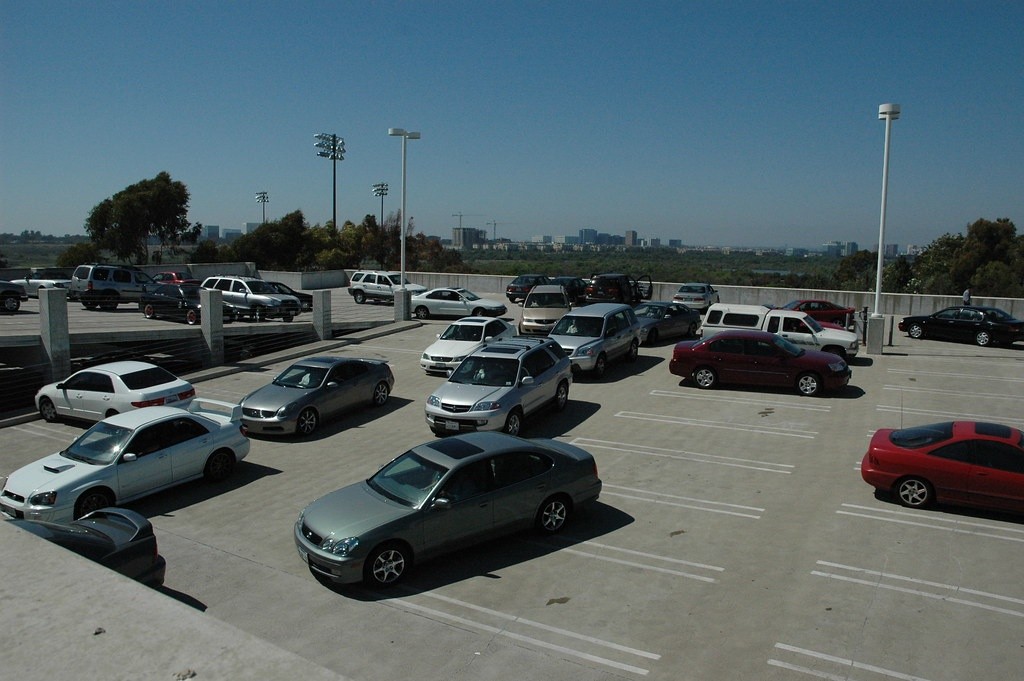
153, 272, 203, 284
668, 331, 852, 397
778, 299, 855, 328
294, 431, 602, 595
0, 355, 395, 591
671, 283, 720, 316
505, 274, 586, 303
138, 284, 240, 325
549, 277, 586, 307
633, 301, 702, 347
266, 281, 313, 313
9, 271, 77, 301
411, 286, 509, 376
861, 421, 1024, 521
898, 306, 1024, 347
0, 280, 29, 312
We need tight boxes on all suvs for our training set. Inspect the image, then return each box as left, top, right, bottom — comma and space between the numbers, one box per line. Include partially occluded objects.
584, 274, 654, 306
696, 303, 859, 364
348, 271, 428, 304
201, 275, 302, 322
70, 263, 158, 311
424, 335, 573, 437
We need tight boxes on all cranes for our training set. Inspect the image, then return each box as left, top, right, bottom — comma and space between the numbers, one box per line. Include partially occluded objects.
486, 220, 518, 242
451, 212, 486, 229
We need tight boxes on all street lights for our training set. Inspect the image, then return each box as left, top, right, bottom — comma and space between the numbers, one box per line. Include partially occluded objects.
314, 133, 346, 230
865, 103, 901, 356
256, 192, 269, 224
388, 127, 421, 321
372, 183, 388, 228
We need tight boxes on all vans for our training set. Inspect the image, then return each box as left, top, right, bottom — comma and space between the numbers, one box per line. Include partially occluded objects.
518, 284, 642, 378
